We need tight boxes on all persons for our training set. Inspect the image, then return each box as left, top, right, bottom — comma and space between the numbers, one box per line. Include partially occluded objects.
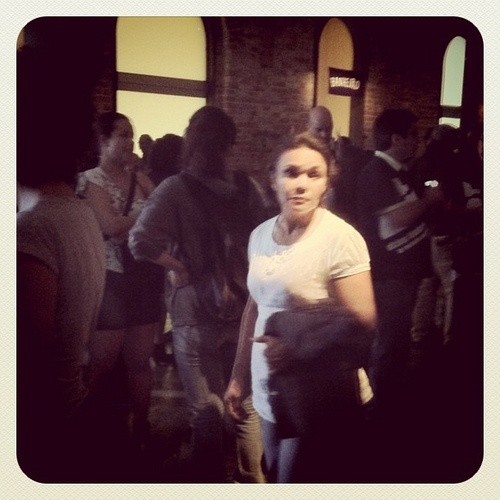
342, 106, 449, 409
147, 131, 185, 192
17, 78, 107, 484
128, 104, 266, 484
406, 96, 483, 358
334, 135, 360, 223
222, 133, 380, 483
298, 102, 344, 213
74, 110, 170, 452
139, 133, 151, 163
